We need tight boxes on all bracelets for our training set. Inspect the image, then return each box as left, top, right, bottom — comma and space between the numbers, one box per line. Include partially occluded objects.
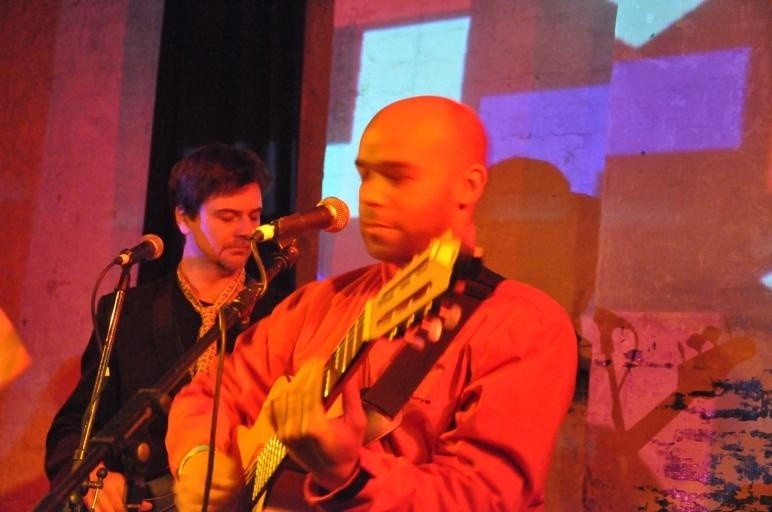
179, 443, 212, 476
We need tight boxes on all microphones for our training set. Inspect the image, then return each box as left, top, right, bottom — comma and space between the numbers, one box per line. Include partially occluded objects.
254, 196, 351, 240
113, 231, 165, 266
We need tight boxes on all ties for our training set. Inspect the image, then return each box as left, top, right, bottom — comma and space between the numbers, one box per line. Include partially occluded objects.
177, 264, 248, 376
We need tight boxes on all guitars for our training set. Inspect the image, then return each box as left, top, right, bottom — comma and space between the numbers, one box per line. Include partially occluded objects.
225, 228, 483, 512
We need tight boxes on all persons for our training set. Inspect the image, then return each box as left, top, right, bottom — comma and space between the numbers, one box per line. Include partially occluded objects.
45, 142, 296, 511
165, 96, 578, 510
